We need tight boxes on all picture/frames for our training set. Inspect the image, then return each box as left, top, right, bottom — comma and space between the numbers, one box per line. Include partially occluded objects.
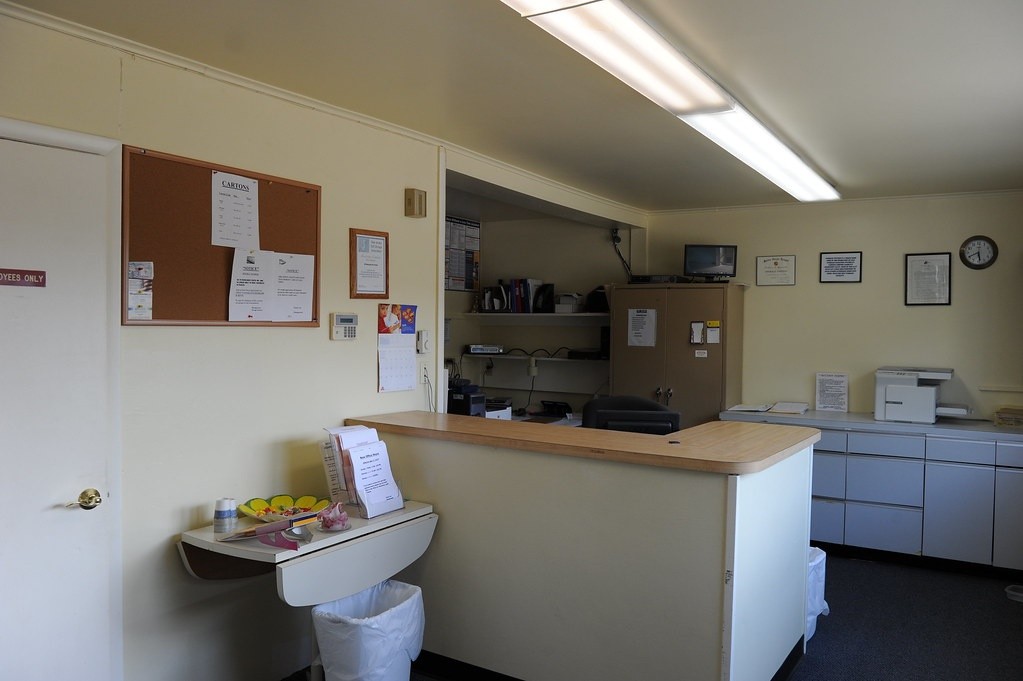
905, 252, 951, 306
349, 227, 389, 300
819, 251, 862, 283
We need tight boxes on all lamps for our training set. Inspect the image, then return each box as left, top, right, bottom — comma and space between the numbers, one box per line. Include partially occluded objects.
502, 0, 842, 203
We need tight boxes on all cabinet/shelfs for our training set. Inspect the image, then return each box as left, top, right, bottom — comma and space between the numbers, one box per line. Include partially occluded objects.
719, 405, 1023, 587
462, 312, 611, 364
608, 282, 744, 433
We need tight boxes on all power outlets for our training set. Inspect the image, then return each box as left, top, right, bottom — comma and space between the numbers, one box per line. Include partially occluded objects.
421, 363, 430, 384
486, 363, 492, 376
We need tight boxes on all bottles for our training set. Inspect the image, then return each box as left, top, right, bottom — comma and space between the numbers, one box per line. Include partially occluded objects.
214, 499, 237, 533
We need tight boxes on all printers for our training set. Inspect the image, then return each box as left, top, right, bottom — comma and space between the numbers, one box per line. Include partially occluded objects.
874, 365, 969, 424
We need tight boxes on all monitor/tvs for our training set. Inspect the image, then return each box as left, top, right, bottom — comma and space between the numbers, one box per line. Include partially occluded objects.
684, 245, 737, 282
595, 410, 679, 434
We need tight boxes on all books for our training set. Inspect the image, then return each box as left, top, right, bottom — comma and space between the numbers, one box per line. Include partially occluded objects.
511, 278, 542, 313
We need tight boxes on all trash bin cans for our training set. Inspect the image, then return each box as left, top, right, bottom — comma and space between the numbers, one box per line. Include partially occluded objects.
807, 547, 826, 641
311, 580, 421, 681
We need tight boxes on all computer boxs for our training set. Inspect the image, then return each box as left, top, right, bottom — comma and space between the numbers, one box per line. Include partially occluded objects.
448, 392, 486, 419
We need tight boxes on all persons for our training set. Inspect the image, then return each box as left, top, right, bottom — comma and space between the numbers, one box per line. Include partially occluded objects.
379, 304, 401, 334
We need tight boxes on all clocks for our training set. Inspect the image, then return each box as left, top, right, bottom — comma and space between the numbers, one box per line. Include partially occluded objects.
959, 235, 999, 270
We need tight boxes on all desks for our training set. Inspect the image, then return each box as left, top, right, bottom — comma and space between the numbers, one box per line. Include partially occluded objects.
175, 493, 440, 681
